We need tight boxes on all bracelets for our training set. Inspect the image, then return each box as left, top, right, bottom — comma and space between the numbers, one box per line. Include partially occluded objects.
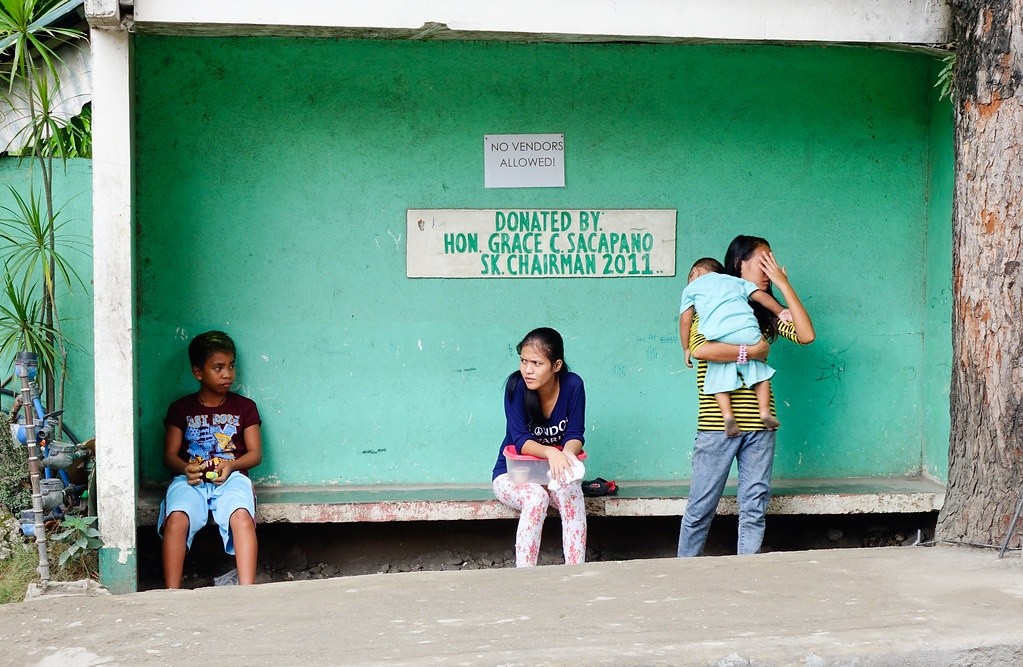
737, 344, 748, 366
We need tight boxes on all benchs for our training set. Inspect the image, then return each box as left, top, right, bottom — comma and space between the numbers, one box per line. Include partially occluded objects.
146, 473, 948, 520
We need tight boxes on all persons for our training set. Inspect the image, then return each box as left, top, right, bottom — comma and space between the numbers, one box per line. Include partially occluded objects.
679, 257, 794, 438
677, 236, 816, 557
157, 331, 262, 588
492, 328, 587, 567
